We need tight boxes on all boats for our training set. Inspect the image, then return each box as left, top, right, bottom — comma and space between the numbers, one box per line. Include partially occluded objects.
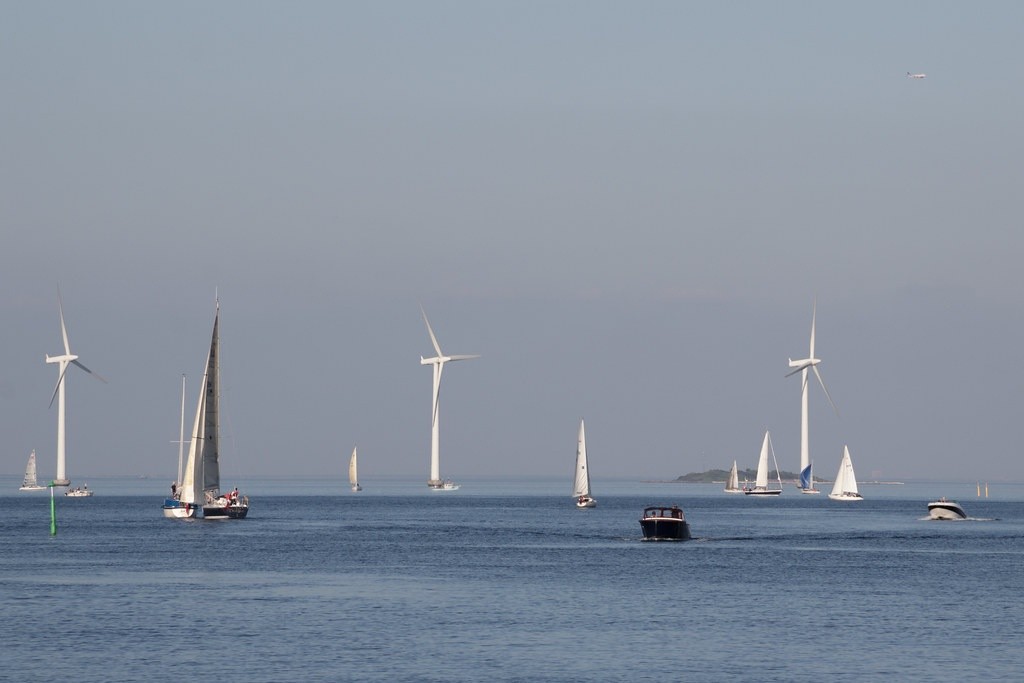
638, 504, 691, 541
431, 480, 462, 491
927, 496, 967, 520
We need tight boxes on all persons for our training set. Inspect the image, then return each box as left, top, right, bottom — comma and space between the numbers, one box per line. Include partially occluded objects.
671, 504, 679, 518
171, 482, 177, 496
230, 487, 239, 505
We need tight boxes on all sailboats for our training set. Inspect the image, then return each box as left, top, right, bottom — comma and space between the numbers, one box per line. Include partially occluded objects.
199, 306, 249, 519
799, 460, 820, 494
161, 373, 206, 519
64, 482, 94, 497
349, 444, 363, 491
572, 416, 597, 508
18, 449, 48, 491
744, 430, 783, 496
828, 444, 864, 501
724, 460, 744, 493
